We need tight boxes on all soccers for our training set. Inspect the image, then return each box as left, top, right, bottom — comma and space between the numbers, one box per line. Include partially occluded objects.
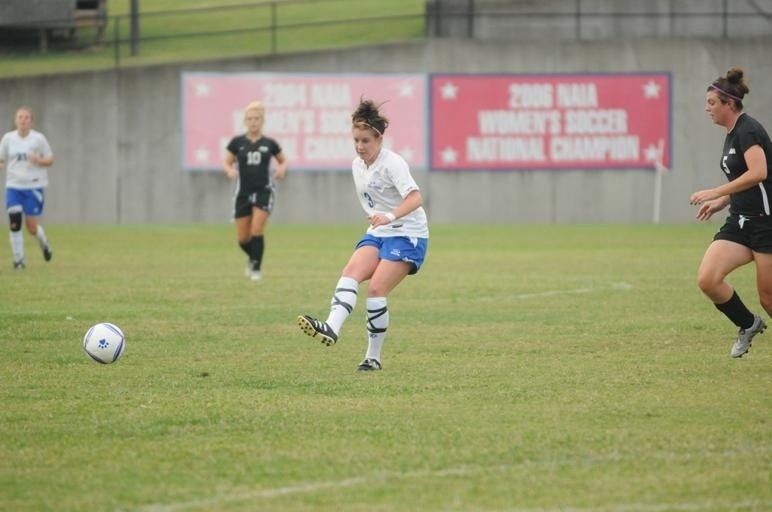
83, 322, 125, 364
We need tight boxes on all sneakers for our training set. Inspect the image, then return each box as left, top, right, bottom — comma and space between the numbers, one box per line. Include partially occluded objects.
730, 314, 768, 359
356, 358, 381, 372
244, 259, 261, 280
13, 261, 25, 271
296, 315, 337, 347
43, 237, 51, 263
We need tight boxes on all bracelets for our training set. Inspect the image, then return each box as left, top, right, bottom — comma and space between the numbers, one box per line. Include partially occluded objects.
384, 212, 397, 222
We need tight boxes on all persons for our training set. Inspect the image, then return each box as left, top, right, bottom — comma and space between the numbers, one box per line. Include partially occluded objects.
223, 102, 286, 282
297, 93, 429, 372
689, 68, 771, 359
0, 106, 54, 270
